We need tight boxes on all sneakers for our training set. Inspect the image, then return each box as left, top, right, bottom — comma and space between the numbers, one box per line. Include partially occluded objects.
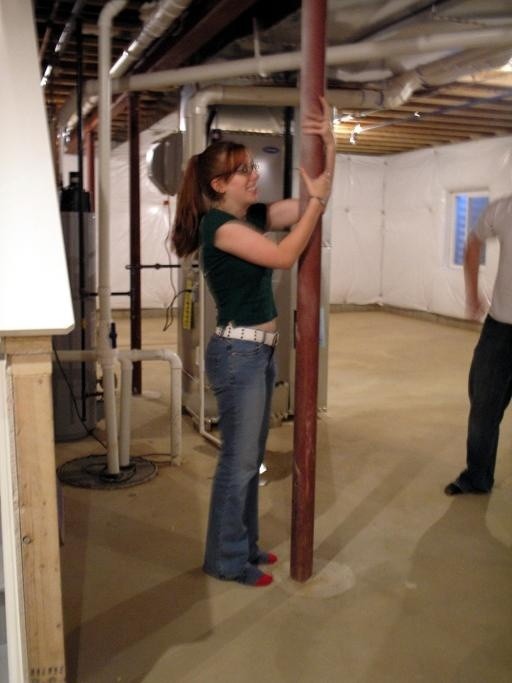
445, 470, 490, 495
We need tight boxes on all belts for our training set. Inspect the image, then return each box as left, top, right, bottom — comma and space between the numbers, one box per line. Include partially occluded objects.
215, 324, 279, 347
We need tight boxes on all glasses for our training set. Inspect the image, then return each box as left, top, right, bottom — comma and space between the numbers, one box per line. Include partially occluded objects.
217, 164, 258, 177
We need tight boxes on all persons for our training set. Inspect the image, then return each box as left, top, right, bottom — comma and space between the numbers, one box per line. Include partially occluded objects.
441, 194, 512, 498
163, 94, 337, 586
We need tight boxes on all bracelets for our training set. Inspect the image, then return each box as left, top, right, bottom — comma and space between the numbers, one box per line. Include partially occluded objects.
308, 195, 328, 207
